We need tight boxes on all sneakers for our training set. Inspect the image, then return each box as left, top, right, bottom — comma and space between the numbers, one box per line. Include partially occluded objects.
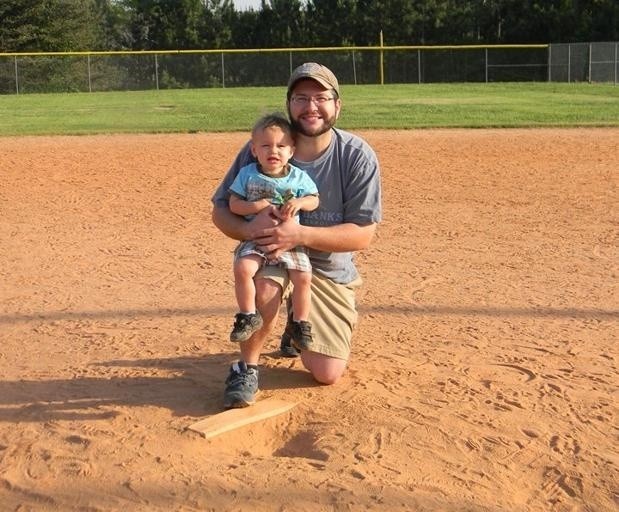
280, 321, 314, 358
223, 360, 260, 406
229, 309, 264, 343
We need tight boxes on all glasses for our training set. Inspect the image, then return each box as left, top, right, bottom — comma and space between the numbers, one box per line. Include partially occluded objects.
290, 95, 336, 103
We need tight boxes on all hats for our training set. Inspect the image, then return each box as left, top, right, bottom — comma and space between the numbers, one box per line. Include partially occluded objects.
289, 62, 338, 94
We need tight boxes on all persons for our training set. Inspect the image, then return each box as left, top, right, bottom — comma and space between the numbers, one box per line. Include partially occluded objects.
229, 110, 322, 352
211, 61, 383, 408
